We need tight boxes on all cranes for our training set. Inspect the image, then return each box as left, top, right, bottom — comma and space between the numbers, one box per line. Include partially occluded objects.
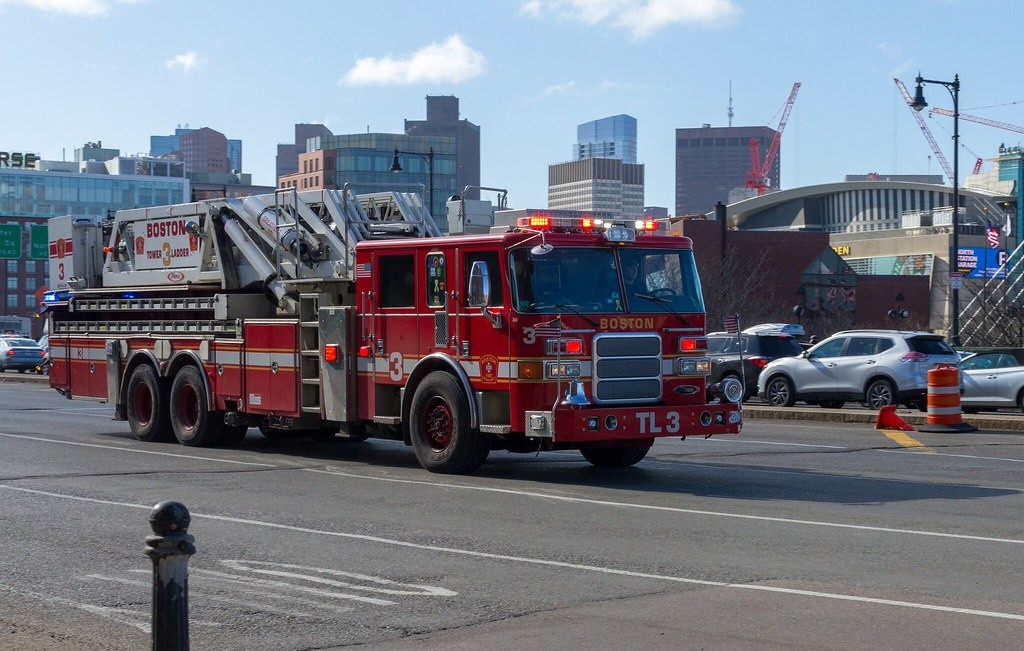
894, 77, 955, 184
925, 98, 1024, 135
745, 81, 802, 193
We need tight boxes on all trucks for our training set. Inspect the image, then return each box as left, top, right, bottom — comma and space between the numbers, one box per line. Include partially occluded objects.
0, 315, 32, 340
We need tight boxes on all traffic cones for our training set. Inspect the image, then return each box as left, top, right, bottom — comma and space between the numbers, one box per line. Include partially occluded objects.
875, 405, 914, 431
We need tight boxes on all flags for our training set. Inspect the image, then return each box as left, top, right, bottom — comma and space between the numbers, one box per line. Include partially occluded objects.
986, 219, 1000, 249
1002, 213, 1011, 236
724, 315, 738, 333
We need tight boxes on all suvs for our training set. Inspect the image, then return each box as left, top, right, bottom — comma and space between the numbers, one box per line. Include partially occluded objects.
702, 322, 813, 405
757, 328, 965, 411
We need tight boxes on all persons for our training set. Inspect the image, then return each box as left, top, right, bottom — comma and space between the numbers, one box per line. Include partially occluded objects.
515, 260, 524, 275
614, 257, 655, 298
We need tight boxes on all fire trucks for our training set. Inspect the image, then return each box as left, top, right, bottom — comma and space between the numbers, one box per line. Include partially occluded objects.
36, 181, 746, 472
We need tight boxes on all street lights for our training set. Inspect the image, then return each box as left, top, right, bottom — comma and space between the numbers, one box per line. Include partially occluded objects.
388, 145, 434, 219
909, 70, 960, 344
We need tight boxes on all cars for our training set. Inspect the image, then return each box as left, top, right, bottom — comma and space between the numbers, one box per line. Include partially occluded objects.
38, 333, 50, 376
963, 347, 1024, 412
0, 336, 45, 373
